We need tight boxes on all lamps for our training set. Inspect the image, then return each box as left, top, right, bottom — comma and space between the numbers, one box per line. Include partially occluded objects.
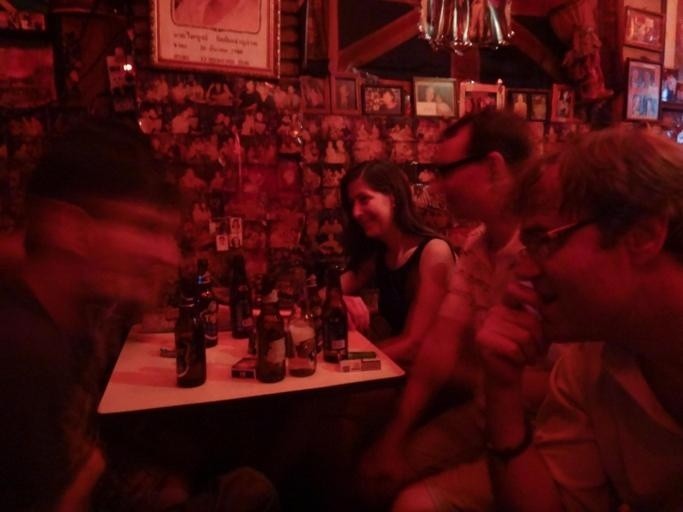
412, 1, 517, 55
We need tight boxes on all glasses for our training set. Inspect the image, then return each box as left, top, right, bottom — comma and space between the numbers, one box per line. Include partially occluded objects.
519, 219, 595, 259
431, 156, 471, 178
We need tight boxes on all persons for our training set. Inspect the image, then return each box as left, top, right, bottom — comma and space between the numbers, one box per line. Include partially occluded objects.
270, 112, 683, 510
144, 71, 571, 119
141, 106, 446, 289
0, 114, 274, 511
630, 68, 676, 116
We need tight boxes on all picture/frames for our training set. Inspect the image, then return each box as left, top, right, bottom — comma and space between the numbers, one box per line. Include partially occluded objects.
292, 73, 579, 122
148, 0, 282, 80
622, 57, 661, 123
622, 4, 667, 51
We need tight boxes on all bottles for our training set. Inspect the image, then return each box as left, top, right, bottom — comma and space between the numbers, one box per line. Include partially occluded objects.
172, 250, 349, 387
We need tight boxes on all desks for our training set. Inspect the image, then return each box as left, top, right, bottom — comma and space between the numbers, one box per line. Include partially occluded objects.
95, 304, 408, 420
